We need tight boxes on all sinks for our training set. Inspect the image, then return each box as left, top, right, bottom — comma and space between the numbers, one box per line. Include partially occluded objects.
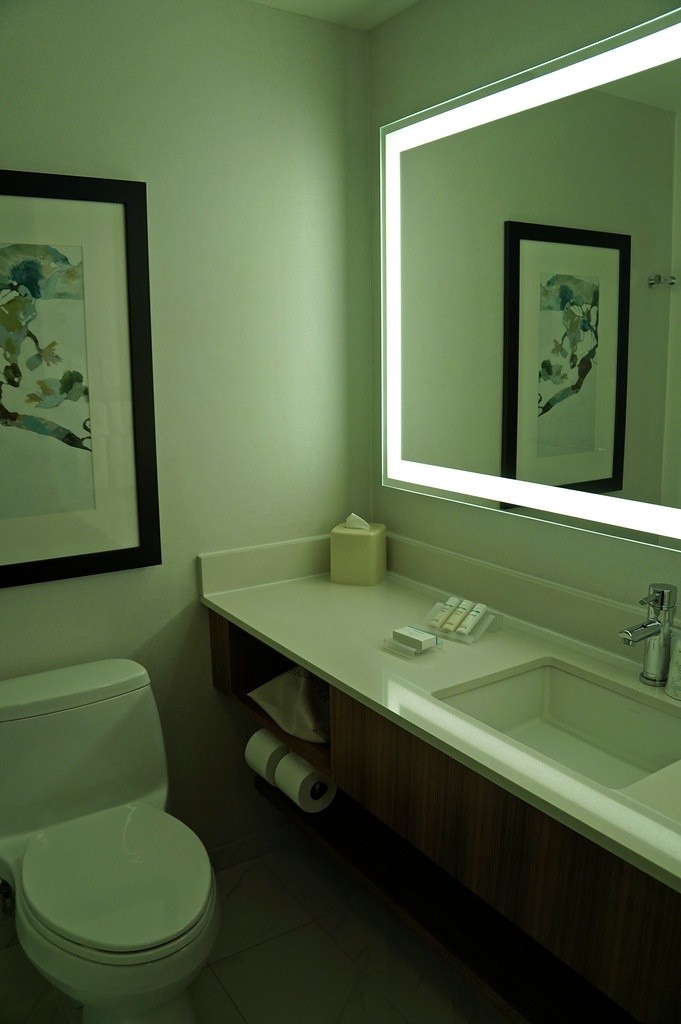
418, 651, 680, 802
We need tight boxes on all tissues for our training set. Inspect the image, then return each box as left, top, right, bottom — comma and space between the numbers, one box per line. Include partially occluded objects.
328, 512, 388, 586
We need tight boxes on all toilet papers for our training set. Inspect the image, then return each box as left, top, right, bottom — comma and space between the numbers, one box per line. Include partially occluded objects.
272, 754, 337, 816
240, 729, 292, 788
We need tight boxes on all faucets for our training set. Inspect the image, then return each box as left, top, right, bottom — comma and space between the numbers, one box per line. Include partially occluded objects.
616, 582, 679, 692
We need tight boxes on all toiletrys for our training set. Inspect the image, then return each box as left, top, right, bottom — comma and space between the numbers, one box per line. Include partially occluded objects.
430, 596, 461, 628
453, 602, 488, 635
439, 598, 472, 632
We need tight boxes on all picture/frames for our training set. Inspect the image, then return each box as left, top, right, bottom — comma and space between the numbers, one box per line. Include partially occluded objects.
499, 220, 633, 512
0, 168, 165, 591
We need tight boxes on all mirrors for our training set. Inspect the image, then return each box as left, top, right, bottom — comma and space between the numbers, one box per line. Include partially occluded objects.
377, 6, 681, 555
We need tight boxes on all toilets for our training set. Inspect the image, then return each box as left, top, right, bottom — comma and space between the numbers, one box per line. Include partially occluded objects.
1, 650, 222, 1024
